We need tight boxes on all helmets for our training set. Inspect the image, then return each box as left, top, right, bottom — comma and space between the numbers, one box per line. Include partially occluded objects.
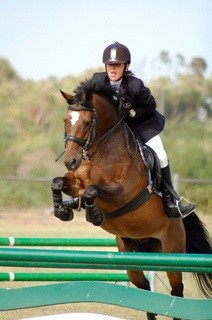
103, 42, 132, 65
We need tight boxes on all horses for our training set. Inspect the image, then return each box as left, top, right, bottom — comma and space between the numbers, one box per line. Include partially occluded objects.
51, 77, 212, 320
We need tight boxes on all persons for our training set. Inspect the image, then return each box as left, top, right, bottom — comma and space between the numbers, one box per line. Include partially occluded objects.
87, 41, 196, 222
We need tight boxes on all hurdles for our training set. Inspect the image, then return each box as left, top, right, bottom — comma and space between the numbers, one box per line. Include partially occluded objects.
0, 234, 212, 320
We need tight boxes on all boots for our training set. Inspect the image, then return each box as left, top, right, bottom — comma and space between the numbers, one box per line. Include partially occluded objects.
160, 162, 195, 221
